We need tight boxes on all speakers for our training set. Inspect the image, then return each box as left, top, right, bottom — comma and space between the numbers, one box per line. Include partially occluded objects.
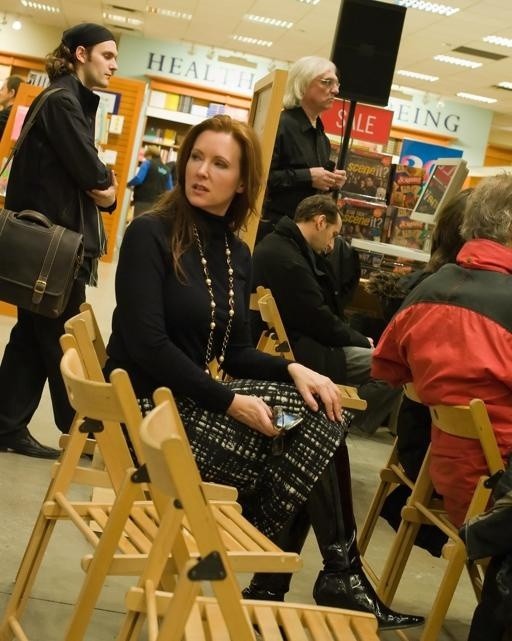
328, 0, 407, 108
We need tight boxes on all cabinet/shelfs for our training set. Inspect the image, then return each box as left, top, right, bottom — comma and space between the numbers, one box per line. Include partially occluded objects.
128, 86, 250, 228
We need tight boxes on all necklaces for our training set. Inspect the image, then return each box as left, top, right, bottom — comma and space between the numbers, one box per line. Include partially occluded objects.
191, 223, 236, 382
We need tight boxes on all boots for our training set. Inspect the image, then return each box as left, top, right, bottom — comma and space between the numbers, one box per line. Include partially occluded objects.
242, 501, 310, 641
309, 431, 424, 630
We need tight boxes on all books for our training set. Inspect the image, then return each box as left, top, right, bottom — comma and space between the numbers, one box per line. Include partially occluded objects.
138, 127, 177, 163
26, 70, 51, 88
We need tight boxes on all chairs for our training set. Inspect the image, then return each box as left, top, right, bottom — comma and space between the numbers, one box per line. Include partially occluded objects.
18, 303, 240, 584
386, 399, 503, 633
2, 336, 302, 641
113, 388, 386, 640
253, 288, 365, 413
355, 380, 462, 615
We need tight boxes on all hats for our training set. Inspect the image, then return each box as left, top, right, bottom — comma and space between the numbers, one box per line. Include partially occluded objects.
62, 23, 116, 53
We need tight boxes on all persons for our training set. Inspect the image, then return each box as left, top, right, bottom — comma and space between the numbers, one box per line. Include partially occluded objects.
253, 54, 348, 247
364, 186, 476, 558
126, 143, 174, 217
251, 195, 403, 435
0, 74, 25, 141
101, 112, 427, 641
0, 21, 119, 459
370, 170, 512, 529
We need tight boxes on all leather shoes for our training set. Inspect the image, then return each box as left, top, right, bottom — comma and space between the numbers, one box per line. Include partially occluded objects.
0, 429, 60, 459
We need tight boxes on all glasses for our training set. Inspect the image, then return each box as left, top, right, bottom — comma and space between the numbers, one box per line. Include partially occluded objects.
317, 78, 340, 87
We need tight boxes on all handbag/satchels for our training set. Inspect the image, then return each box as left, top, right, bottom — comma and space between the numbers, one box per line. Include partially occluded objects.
0, 208, 85, 318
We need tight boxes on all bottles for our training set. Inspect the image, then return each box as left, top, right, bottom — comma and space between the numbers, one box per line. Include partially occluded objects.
423, 234, 432, 253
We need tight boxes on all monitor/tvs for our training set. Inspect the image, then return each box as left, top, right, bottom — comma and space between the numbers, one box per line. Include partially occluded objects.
406, 154, 472, 231
